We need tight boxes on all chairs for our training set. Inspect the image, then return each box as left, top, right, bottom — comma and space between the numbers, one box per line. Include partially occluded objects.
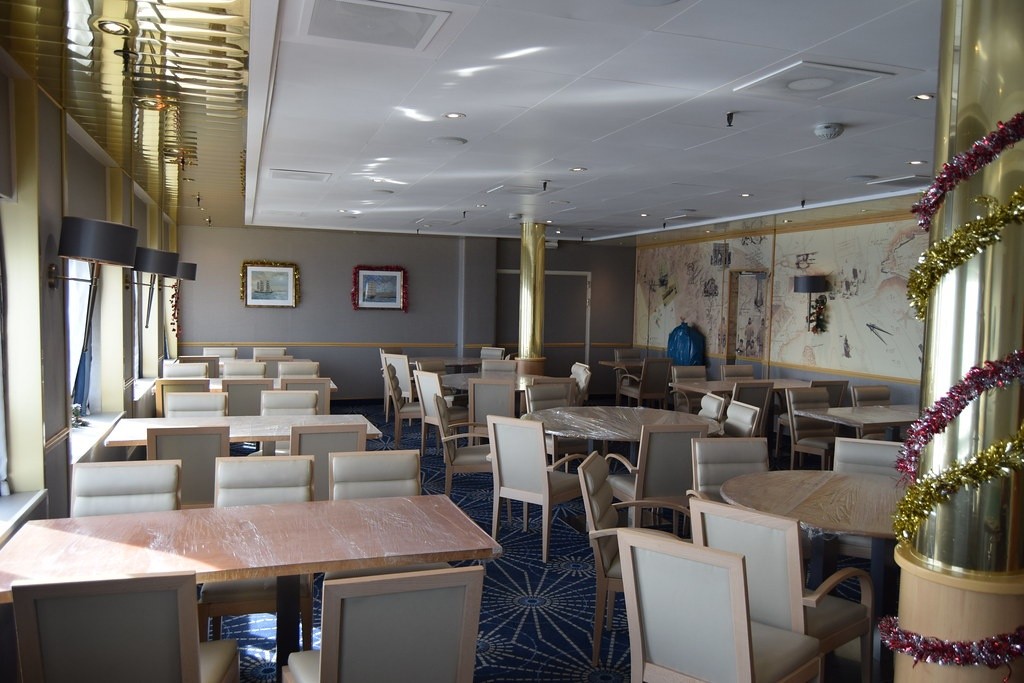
164, 363, 209, 378
290, 423, 368, 502
179, 356, 219, 377
222, 362, 266, 378
222, 379, 274, 416
281, 377, 330, 415
165, 391, 229, 418
248, 390, 319, 457
323, 450, 451, 586
203, 347, 238, 359
254, 347, 287, 358
277, 362, 320, 378
380, 340, 908, 683
282, 565, 487, 683
146, 426, 230, 510
199, 454, 317, 651
256, 357, 294, 378
10, 569, 240, 683
70, 458, 183, 518
156, 379, 210, 418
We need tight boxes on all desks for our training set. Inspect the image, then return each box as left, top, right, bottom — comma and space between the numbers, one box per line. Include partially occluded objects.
599, 357, 674, 407
175, 359, 314, 378
793, 404, 921, 444
408, 357, 506, 395
105, 414, 383, 456
521, 406, 721, 535
440, 371, 558, 445
151, 376, 339, 413
669, 377, 811, 469
720, 471, 906, 683
0, 494, 504, 683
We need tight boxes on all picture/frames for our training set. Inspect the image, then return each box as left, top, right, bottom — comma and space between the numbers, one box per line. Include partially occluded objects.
244, 264, 296, 309
356, 269, 404, 311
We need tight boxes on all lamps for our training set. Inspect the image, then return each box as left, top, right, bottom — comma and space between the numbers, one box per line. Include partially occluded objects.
159, 260, 198, 296
124, 246, 179, 329
49, 215, 139, 352
793, 274, 834, 333
